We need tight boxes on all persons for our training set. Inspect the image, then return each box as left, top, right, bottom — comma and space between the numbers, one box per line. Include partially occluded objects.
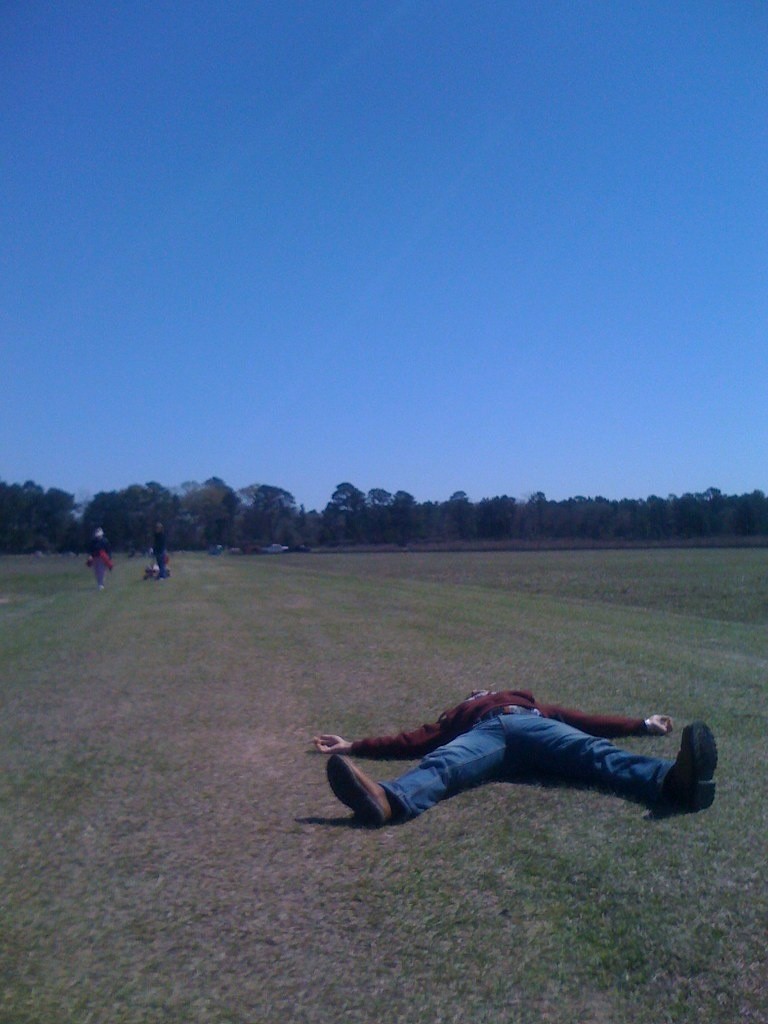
30, 523, 171, 592
312, 688, 718, 830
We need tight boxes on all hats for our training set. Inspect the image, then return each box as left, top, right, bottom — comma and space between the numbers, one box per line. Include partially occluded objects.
95, 527, 103, 538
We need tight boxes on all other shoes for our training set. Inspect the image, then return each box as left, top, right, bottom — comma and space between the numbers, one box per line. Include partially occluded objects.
327, 755, 392, 828
98, 585, 104, 592
672, 721, 718, 810
